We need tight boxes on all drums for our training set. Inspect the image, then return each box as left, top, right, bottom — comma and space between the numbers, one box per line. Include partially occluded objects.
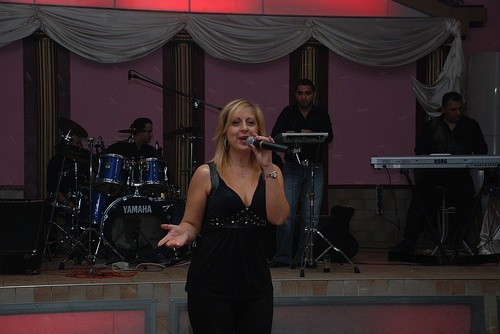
84, 153, 187, 262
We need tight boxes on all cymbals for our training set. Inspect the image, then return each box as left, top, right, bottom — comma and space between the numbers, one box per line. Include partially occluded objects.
118, 129, 146, 134
170, 127, 198, 135
56, 117, 89, 139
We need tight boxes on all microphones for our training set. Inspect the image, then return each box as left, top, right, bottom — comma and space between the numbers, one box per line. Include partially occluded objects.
128, 70, 131, 80
245, 136, 289, 153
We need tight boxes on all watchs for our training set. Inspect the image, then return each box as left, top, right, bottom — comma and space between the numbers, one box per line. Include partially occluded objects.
263, 171, 277, 181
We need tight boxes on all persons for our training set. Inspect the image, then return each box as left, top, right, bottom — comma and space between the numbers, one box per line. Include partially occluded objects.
270, 78, 334, 268
108, 117, 162, 245
157, 98, 290, 334
46, 129, 82, 240
392, 91, 488, 251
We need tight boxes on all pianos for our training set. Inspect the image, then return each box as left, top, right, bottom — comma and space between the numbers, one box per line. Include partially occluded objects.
370, 154, 500, 263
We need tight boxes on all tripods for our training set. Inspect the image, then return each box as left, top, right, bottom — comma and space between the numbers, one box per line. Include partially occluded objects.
39, 135, 125, 276
275, 130, 361, 278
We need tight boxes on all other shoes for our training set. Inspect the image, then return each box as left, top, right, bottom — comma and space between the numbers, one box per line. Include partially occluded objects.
299, 262, 317, 268
385, 240, 414, 251
270, 261, 288, 268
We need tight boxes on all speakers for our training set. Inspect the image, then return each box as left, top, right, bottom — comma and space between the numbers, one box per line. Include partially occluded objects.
0, 198, 44, 274
